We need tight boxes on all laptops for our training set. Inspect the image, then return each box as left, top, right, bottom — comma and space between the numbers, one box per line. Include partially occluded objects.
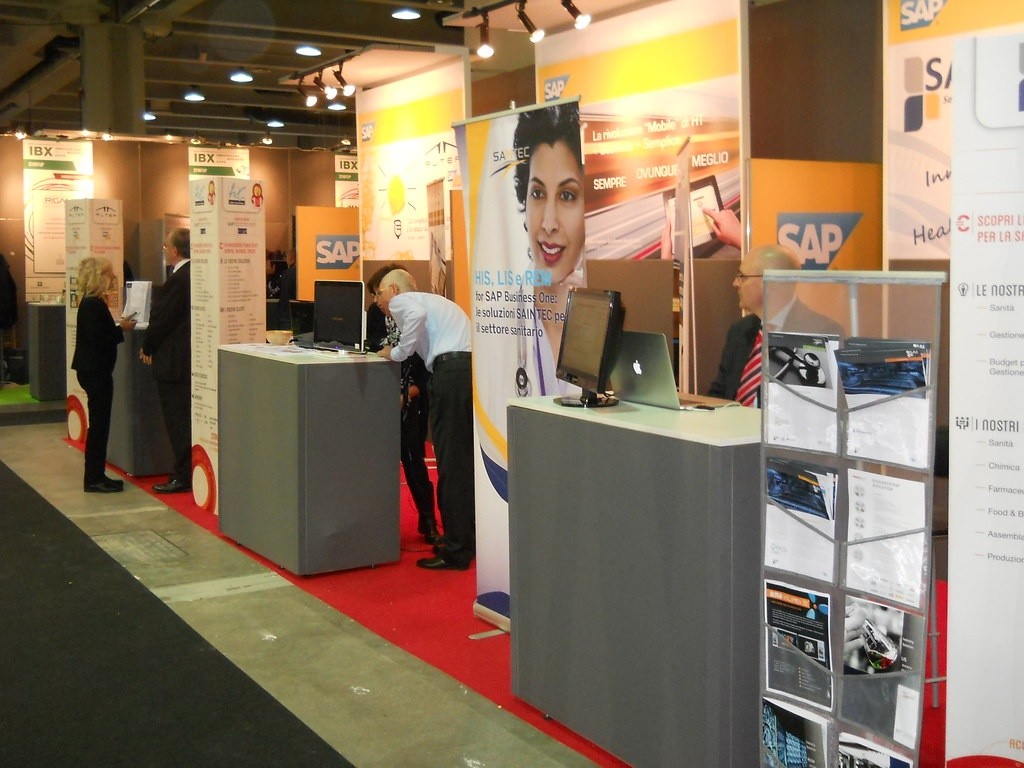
609, 331, 739, 411
289, 300, 317, 349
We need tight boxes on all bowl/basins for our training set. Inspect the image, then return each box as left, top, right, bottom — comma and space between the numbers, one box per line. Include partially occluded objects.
265, 331, 292, 344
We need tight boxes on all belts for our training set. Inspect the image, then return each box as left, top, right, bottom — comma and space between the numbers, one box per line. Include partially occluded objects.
433, 352, 471, 369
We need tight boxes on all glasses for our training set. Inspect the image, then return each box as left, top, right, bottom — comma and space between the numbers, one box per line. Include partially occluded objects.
371, 284, 392, 299
736, 271, 763, 281
163, 244, 174, 251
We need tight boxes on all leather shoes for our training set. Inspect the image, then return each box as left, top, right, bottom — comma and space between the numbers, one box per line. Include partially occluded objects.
418, 555, 470, 572
418, 525, 440, 543
152, 474, 194, 493
433, 543, 448, 554
84, 475, 123, 492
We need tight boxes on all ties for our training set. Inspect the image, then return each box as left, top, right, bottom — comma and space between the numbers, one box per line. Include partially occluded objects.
736, 324, 776, 406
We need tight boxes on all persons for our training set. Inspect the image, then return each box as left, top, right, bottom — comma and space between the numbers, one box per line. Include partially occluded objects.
659, 209, 744, 260
71, 257, 137, 493
139, 228, 193, 494
700, 248, 845, 408
363, 264, 477, 571
508, 107, 585, 398
844, 610, 871, 654
266, 247, 296, 299
1, 253, 18, 329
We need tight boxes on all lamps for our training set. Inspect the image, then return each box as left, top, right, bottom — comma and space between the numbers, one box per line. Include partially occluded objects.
475, 15, 494, 58
141, 8, 422, 146
559, 0, 592, 29
515, 3, 544, 42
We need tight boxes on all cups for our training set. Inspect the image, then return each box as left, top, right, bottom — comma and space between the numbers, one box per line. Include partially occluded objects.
55, 296, 64, 303
39, 294, 49, 303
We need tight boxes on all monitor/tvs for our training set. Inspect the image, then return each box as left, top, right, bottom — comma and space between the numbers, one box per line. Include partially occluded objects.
312, 281, 366, 357
553, 288, 624, 409
